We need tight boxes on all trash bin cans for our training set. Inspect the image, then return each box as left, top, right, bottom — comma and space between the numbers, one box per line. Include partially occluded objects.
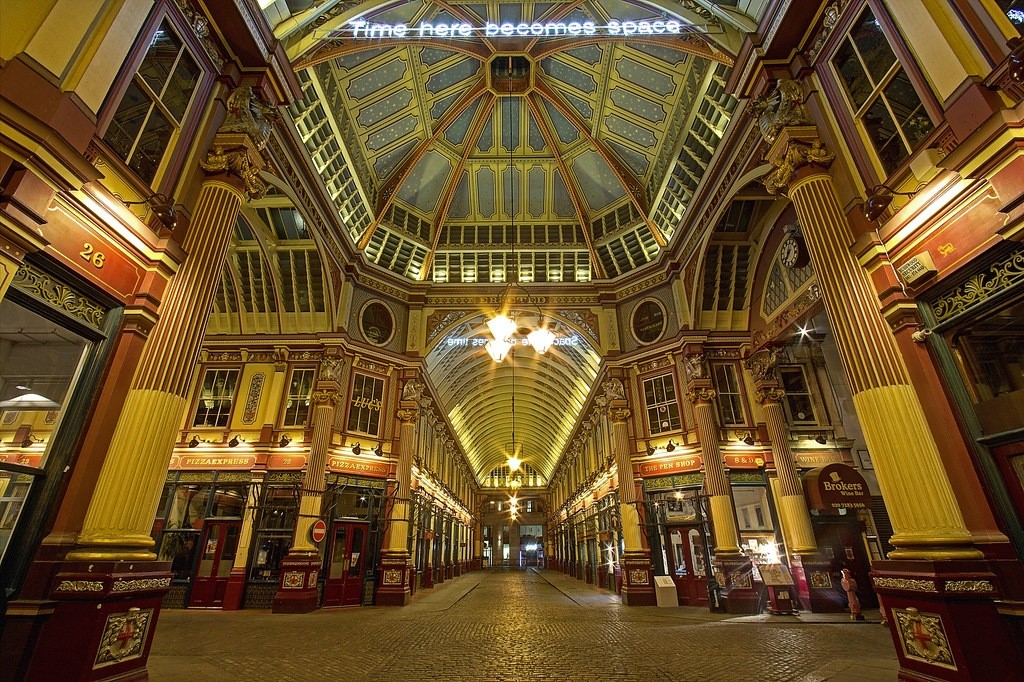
653, 576, 679, 608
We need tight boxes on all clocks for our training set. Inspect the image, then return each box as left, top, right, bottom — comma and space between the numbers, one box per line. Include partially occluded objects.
780, 236, 808, 269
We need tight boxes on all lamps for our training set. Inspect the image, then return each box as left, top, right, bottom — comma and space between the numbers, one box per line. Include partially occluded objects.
667, 439, 679, 452
862, 185, 919, 223
20, 435, 44, 448
486, 57, 556, 364
646, 444, 657, 455
371, 443, 383, 456
504, 347, 523, 520
229, 434, 245, 448
189, 435, 205, 447
808, 431, 826, 445
123, 194, 178, 232
280, 434, 292, 447
351, 442, 361, 455
739, 432, 754, 445
